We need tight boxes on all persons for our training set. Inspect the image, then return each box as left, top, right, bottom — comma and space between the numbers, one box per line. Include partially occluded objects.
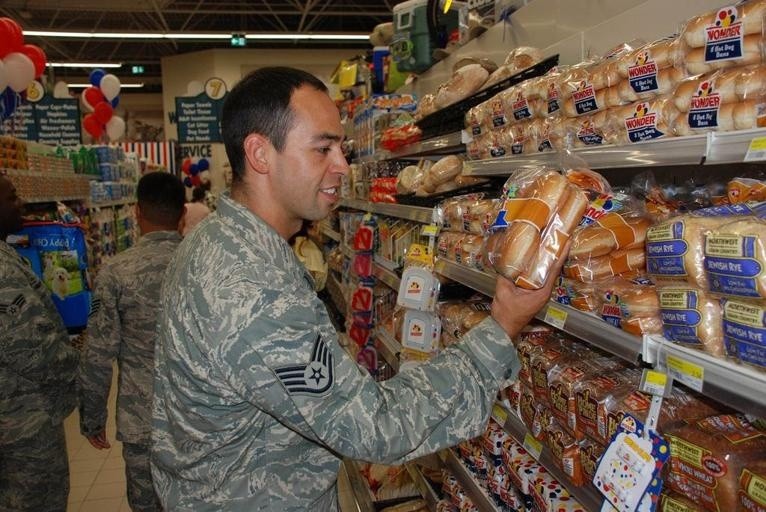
285, 218, 330, 296
198, 175, 219, 214
0, 170, 83, 511
78, 172, 190, 512
147, 63, 574, 510
184, 185, 212, 237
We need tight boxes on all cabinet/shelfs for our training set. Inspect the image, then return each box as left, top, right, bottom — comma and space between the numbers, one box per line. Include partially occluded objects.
0, 137, 142, 348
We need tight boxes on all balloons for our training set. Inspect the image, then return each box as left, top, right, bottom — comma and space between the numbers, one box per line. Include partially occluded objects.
1, 16, 47, 127
181, 155, 212, 201
81, 67, 127, 142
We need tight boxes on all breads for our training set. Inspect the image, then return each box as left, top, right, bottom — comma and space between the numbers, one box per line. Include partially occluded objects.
461, 0, 765, 161
404, 44, 543, 121
434, 163, 765, 379
384, 284, 484, 359
431, 292, 765, 510
392, 157, 477, 195
